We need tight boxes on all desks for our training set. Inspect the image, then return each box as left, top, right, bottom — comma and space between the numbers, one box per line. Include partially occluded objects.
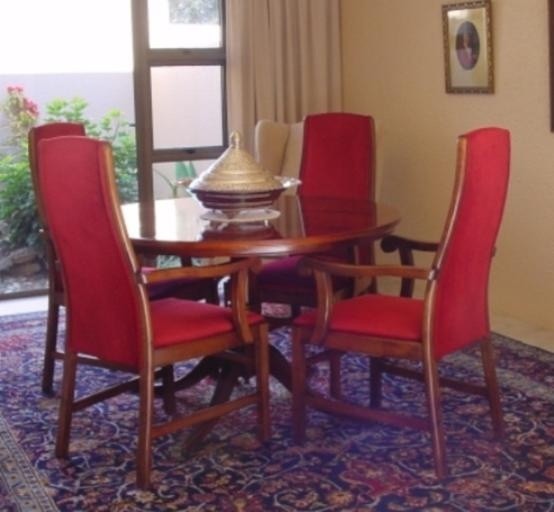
111, 195, 442, 456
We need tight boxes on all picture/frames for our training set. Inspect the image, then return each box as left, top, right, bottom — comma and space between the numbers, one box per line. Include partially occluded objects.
441, 0, 494, 94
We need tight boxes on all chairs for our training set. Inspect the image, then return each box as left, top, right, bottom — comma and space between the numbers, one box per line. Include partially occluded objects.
289, 128, 511, 483
34, 137, 272, 489
224, 113, 376, 397
29, 123, 174, 413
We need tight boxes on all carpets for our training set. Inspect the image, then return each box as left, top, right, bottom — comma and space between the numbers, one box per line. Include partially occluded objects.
0, 305, 554, 512
